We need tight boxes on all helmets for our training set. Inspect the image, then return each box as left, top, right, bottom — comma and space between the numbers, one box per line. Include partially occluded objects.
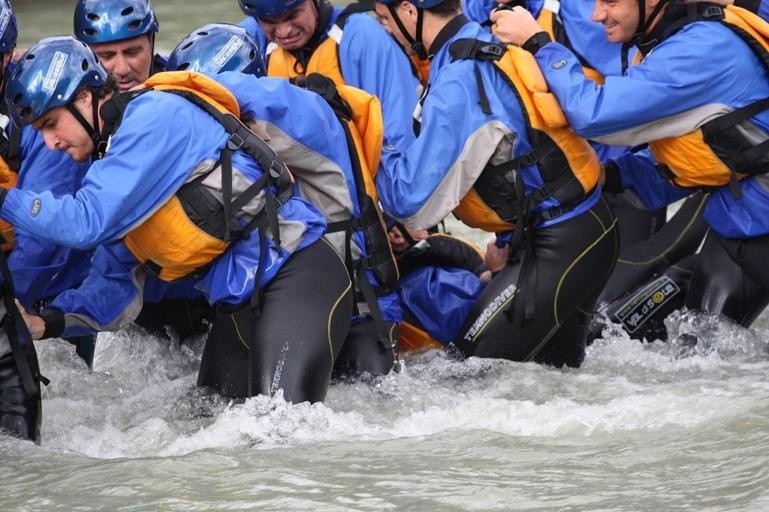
237, 0, 305, 17
0, 0, 18, 56
5, 36, 110, 129
167, 23, 266, 81
74, 1, 160, 44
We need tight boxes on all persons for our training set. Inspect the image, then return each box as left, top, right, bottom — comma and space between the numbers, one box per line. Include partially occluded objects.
0, 0, 769, 444
